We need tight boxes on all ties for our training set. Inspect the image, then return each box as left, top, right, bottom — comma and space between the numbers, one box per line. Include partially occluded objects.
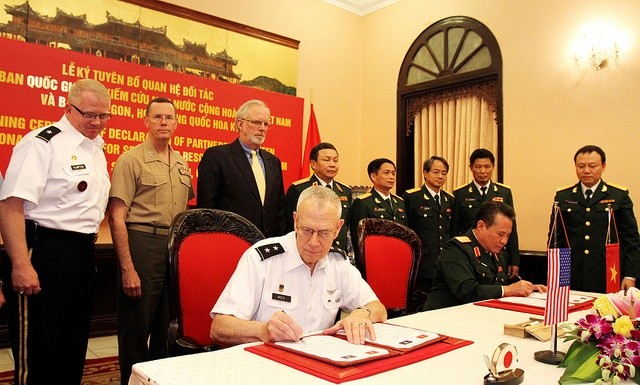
251, 149, 266, 207
586, 190, 594, 202
386, 199, 393, 211
481, 188, 488, 200
326, 185, 332, 190
435, 195, 442, 208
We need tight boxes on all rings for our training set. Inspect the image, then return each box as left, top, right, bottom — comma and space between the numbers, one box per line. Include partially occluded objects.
359, 323, 367, 326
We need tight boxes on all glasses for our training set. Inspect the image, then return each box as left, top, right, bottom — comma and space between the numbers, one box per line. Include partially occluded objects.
430, 169, 449, 178
296, 220, 337, 240
70, 104, 112, 122
148, 116, 174, 123
241, 119, 271, 129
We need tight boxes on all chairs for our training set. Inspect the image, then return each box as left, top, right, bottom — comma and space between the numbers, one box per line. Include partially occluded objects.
166, 207, 267, 355
358, 217, 425, 319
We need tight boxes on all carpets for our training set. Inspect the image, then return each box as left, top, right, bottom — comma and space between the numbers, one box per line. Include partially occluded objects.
0, 359, 120, 385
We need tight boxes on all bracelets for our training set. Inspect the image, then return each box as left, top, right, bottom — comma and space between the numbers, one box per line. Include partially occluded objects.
357, 307, 375, 320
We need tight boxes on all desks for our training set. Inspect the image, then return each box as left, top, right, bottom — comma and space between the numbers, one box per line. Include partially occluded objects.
127, 287, 639, 385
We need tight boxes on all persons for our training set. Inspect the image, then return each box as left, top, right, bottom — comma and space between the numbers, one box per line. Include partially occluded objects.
1, 79, 112, 385
452, 149, 520, 280
197, 100, 285, 241
350, 158, 408, 271
402, 156, 456, 312
285, 143, 352, 256
546, 145, 640, 296
209, 185, 388, 345
108, 98, 195, 385
421, 201, 547, 312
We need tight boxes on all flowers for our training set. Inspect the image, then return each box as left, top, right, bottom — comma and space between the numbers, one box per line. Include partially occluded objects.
557, 288, 640, 385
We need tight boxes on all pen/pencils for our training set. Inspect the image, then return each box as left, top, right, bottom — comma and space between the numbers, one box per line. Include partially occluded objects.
283, 310, 306, 344
516, 273, 534, 294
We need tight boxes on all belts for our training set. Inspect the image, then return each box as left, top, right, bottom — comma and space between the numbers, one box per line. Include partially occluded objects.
127, 223, 170, 237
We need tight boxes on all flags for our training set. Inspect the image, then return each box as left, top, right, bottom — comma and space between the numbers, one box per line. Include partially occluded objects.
298, 103, 322, 180
544, 247, 572, 326
604, 243, 621, 294
